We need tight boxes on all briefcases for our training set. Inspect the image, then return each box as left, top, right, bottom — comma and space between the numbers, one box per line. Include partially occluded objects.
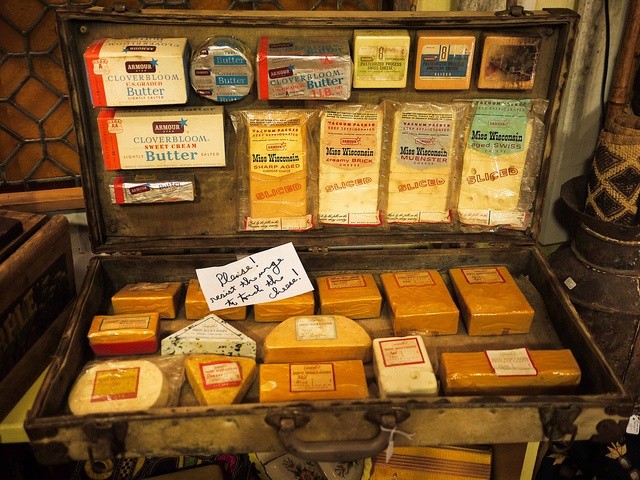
24, 5, 639, 461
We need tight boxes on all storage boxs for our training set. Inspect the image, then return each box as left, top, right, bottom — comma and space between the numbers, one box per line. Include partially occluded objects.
1, 209, 78, 422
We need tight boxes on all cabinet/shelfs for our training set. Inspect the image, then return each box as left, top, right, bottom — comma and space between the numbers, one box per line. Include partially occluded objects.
1, 363, 541, 480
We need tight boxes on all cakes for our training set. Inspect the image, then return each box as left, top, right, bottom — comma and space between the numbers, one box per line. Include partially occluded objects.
160, 312, 258, 358
184, 354, 258, 407
372, 334, 439, 399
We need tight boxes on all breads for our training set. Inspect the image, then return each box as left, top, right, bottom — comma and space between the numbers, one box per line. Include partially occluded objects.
111, 281, 184, 320
317, 106, 384, 228
253, 290, 315, 323
68, 359, 170, 417
184, 279, 247, 321
261, 314, 372, 365
379, 270, 460, 337
440, 348, 582, 395
456, 118, 533, 225
386, 108, 456, 223
448, 266, 536, 336
88, 312, 160, 356
313, 274, 382, 320
245, 113, 311, 231
